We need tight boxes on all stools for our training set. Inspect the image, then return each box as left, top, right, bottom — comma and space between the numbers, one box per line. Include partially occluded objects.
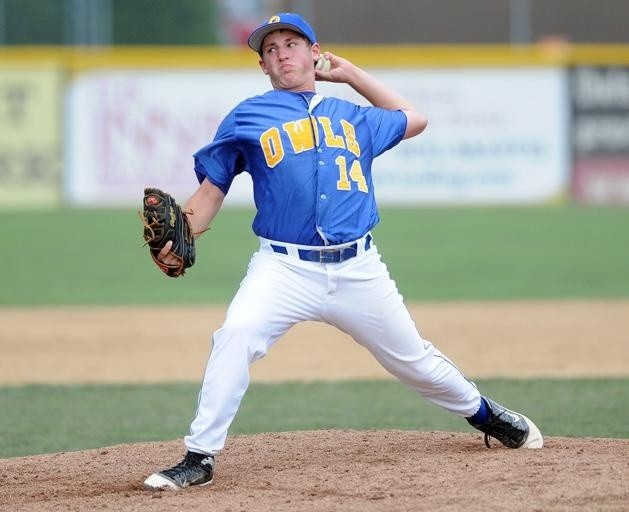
271, 235, 371, 264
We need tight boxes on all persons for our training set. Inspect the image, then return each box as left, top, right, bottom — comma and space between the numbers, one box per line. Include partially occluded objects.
138, 10, 547, 494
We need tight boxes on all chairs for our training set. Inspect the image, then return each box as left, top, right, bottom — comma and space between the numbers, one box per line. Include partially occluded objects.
143, 452, 214, 491
468, 396, 544, 449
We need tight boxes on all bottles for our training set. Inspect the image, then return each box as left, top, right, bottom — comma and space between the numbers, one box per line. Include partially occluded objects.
247, 12, 316, 52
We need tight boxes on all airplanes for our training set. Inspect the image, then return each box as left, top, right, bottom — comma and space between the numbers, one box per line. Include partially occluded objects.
142, 188, 196, 276
